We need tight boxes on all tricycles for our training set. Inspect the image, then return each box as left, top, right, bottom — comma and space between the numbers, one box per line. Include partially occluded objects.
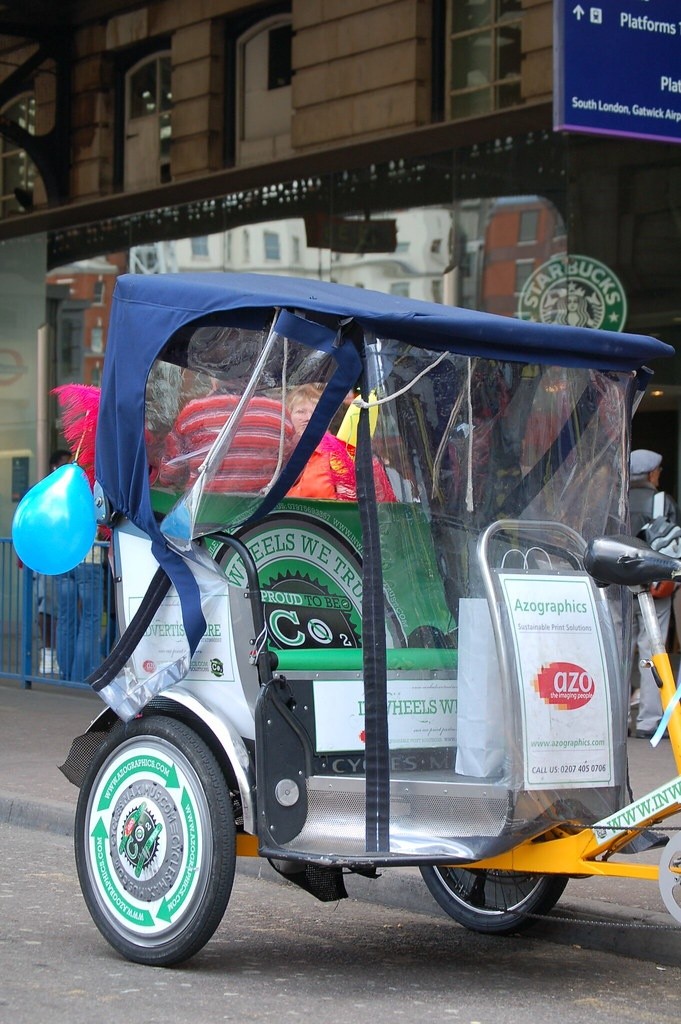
57, 269, 681, 977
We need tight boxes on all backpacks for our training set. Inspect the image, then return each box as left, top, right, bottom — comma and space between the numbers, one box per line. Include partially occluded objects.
635, 491, 681, 559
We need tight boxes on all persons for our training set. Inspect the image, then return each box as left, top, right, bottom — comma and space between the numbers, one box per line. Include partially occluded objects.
158, 355, 414, 502
18, 450, 118, 684
612, 449, 677, 536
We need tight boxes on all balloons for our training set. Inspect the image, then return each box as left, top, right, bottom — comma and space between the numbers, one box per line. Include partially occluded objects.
11, 464, 96, 575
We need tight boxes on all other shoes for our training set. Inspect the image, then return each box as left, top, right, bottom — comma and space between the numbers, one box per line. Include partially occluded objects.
635, 725, 671, 739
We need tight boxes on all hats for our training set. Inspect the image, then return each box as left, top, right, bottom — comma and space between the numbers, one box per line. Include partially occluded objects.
629, 449, 662, 475
